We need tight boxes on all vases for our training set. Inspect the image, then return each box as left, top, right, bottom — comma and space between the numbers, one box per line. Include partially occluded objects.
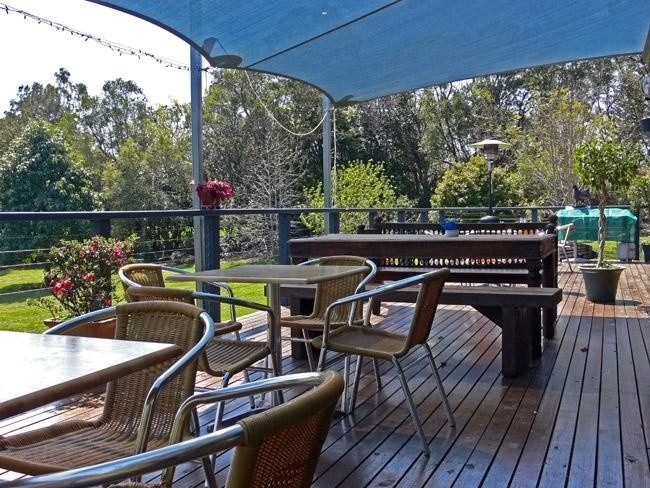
196, 185, 221, 209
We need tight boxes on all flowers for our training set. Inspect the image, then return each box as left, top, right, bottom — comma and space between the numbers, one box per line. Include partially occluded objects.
206, 180, 233, 201
42, 227, 141, 317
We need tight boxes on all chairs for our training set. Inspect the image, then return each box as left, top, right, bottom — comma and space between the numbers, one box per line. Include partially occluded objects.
0, 370, 344, 488
126, 285, 277, 432
0, 300, 216, 488
118, 262, 243, 341
314, 268, 455, 457
296, 254, 378, 371
555, 222, 574, 272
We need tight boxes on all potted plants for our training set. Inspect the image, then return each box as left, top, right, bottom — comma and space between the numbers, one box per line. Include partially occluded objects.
574, 140, 639, 302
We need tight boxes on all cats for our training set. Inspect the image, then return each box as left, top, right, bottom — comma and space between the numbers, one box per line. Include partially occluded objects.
572, 185, 591, 208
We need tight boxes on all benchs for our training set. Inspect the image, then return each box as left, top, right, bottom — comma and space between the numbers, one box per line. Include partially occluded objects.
356, 216, 558, 318
263, 283, 563, 377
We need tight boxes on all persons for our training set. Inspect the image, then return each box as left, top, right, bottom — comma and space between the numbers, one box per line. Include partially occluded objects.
545, 214, 575, 235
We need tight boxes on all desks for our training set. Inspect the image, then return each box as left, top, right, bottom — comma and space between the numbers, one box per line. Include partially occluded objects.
288, 234, 557, 358
1, 328, 184, 422
166, 264, 371, 406
